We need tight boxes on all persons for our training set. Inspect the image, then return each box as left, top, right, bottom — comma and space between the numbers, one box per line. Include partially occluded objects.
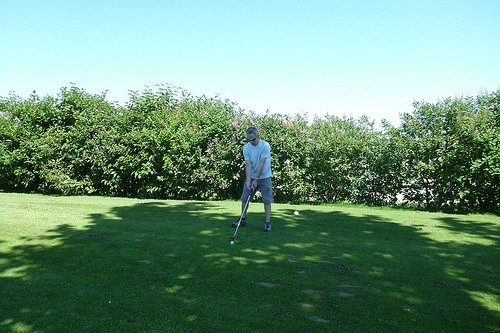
231, 126, 274, 233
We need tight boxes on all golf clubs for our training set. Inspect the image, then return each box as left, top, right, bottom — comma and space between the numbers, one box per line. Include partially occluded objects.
227, 182, 255, 242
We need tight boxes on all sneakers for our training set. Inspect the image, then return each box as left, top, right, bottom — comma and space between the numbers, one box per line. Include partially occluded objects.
264, 222, 271, 232
232, 218, 247, 227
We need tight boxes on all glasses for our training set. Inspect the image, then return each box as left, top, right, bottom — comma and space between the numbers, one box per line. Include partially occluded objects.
247, 137, 258, 142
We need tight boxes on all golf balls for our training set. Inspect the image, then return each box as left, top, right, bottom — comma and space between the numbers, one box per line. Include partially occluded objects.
293, 210, 300, 215
229, 240, 234, 246
